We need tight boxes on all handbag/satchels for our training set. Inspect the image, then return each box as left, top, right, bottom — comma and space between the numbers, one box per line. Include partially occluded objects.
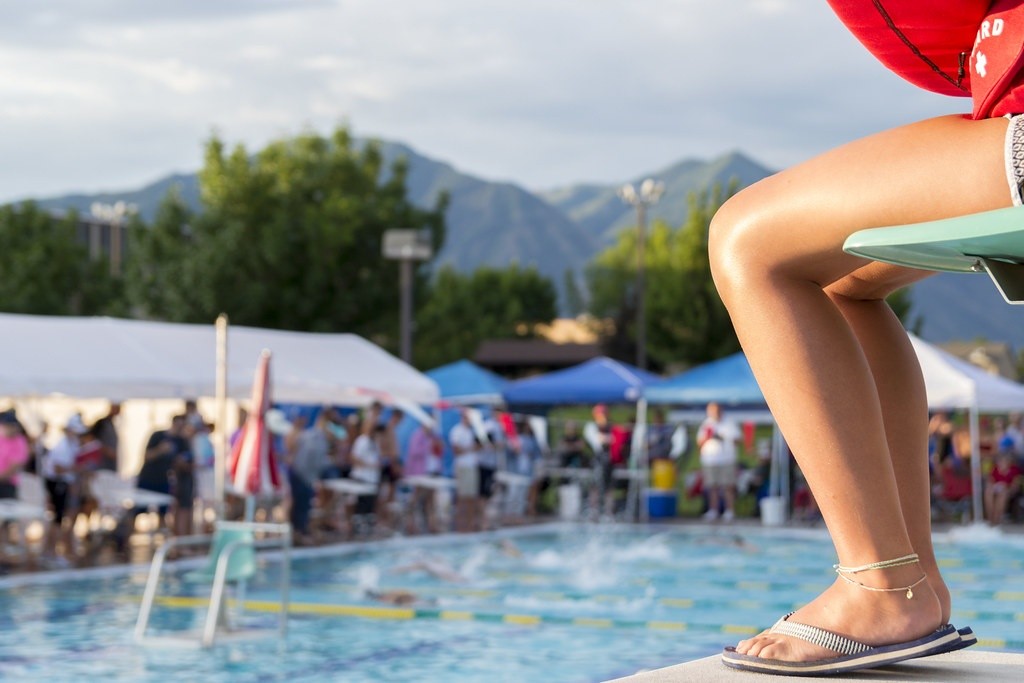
968, 0, 1024, 122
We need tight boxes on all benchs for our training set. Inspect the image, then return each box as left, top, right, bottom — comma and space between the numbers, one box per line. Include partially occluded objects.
547, 467, 648, 519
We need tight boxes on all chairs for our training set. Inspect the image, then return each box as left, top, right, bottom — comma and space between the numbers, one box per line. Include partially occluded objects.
183, 527, 256, 632
841, 201, 1023, 305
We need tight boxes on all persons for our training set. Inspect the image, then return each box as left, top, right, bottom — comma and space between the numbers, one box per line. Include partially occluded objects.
708, 0, 1024, 674
451, 402, 1024, 523
0, 392, 444, 563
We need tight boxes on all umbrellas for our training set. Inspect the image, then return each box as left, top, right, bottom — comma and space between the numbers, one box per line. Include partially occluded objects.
225, 349, 282, 520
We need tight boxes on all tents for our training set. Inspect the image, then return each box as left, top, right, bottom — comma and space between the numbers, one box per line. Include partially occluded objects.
384, 358, 513, 469
440, 356, 667, 522
780, 330, 1024, 525
1, 310, 446, 530
631, 348, 781, 524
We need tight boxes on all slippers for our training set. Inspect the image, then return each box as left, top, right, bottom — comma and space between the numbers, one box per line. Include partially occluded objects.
722, 608, 977, 676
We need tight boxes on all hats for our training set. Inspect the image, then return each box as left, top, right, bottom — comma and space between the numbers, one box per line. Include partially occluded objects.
61, 413, 88, 435
